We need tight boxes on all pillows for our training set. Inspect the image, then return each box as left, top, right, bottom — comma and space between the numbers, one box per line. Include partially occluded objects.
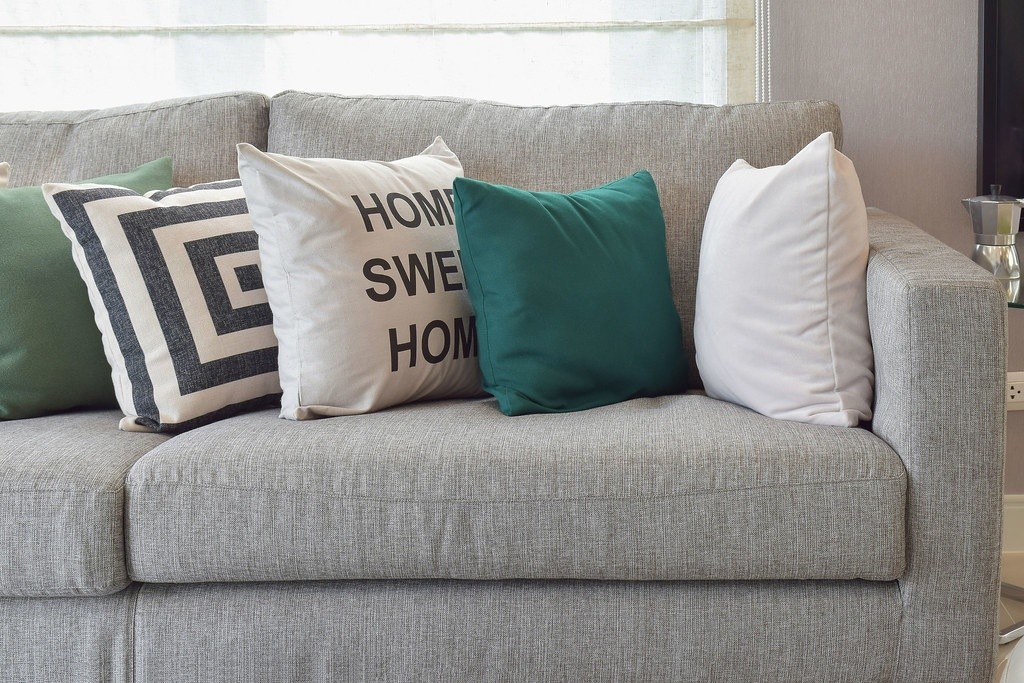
265, 90, 844, 391
236, 135, 490, 421
40, 178, 283, 433
693, 130, 875, 428
0, 154, 174, 422
0, 91, 269, 187
452, 168, 688, 418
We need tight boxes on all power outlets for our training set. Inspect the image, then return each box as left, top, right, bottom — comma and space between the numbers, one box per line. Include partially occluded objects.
1007, 371, 1024, 411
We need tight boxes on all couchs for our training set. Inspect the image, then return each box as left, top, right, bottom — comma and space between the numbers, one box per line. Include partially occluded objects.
0, 204, 1008, 683
